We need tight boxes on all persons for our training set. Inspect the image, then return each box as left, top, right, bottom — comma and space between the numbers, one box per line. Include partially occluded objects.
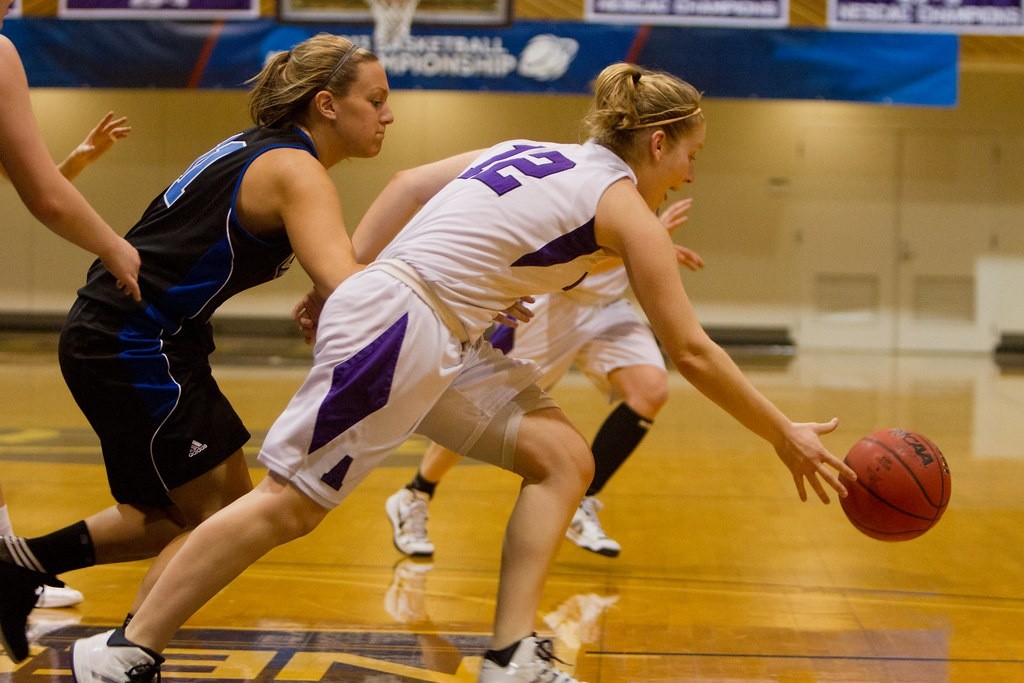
71, 60, 863, 683
0, 0, 708, 668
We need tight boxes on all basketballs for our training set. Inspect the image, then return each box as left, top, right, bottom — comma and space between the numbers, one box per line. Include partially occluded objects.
837, 428, 952, 543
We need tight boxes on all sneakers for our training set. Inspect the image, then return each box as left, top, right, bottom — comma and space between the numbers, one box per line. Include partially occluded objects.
565, 494, 621, 557
479, 632, 579, 683
385, 486, 435, 556
543, 587, 620, 649
69, 614, 164, 683
0, 536, 65, 664
35, 578, 84, 607
384, 558, 434, 623
